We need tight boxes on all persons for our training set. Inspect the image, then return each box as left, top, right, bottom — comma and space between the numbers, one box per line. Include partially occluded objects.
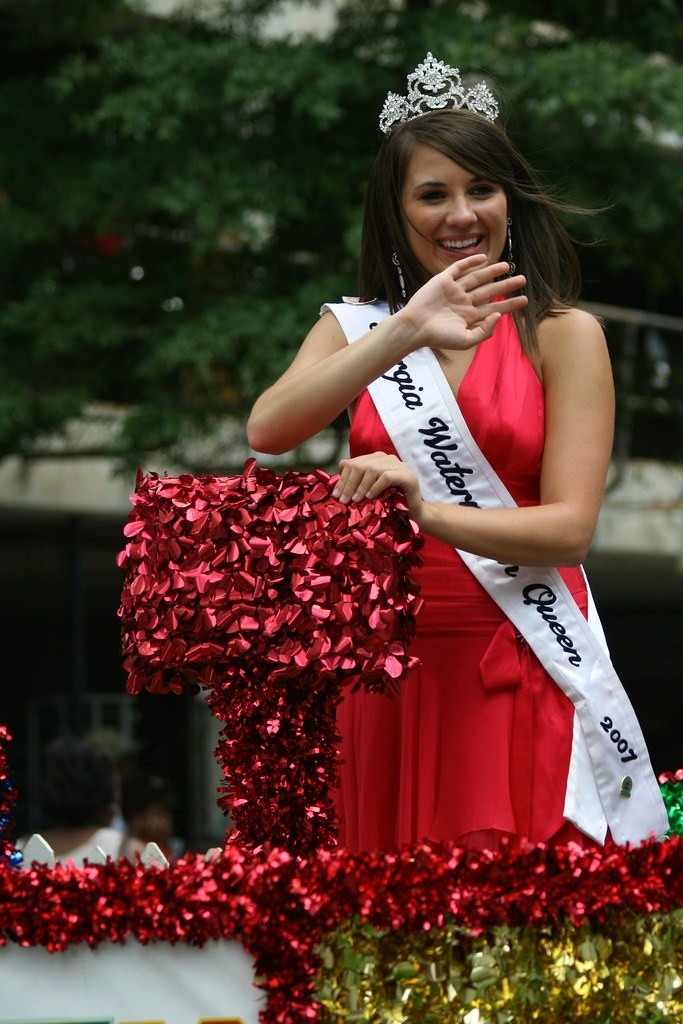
244, 54, 617, 852
14, 738, 155, 872
